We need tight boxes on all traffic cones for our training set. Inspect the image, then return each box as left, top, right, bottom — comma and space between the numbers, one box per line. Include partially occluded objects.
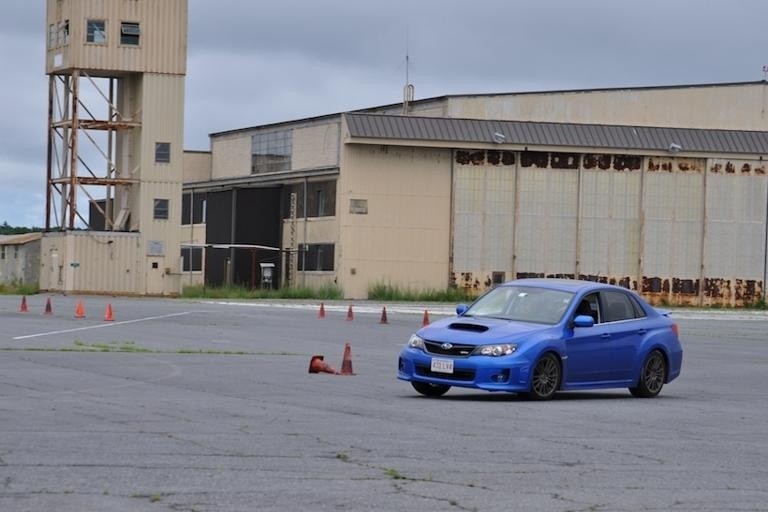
346, 305, 353, 321
43, 297, 53, 315
75, 300, 85, 318
104, 304, 113, 321
421, 310, 429, 327
317, 303, 325, 320
340, 343, 356, 376
380, 307, 389, 324
309, 356, 341, 375
19, 296, 29, 312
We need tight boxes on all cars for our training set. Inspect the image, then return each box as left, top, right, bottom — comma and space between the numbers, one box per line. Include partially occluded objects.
396, 278, 683, 397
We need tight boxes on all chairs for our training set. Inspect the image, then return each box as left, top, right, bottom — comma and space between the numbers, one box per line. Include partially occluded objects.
576, 299, 626, 322
